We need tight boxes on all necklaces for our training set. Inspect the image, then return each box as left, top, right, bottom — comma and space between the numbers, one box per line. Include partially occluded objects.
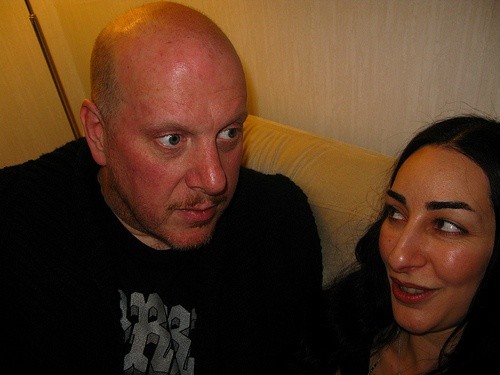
396, 338, 421, 375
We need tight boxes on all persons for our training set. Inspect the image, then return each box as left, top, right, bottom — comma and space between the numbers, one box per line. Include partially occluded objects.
0, 2, 324, 375
323, 115, 500, 375
